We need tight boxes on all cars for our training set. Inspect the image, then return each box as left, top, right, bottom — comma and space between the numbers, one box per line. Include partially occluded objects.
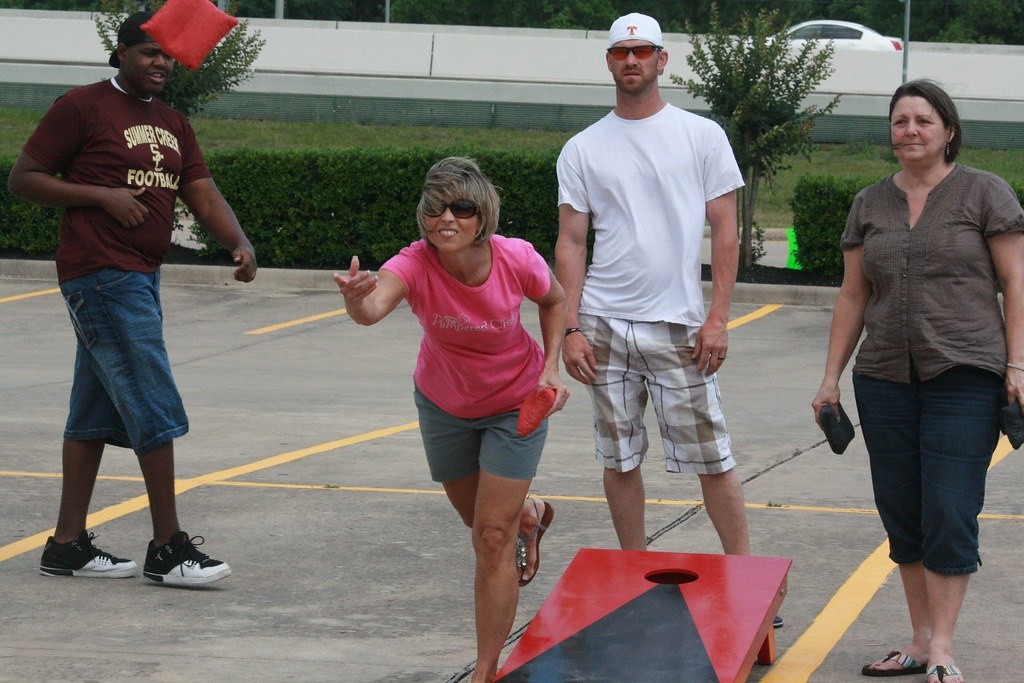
762, 19, 905, 50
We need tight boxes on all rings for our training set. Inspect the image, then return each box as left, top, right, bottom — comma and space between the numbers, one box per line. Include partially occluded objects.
718, 356, 724, 361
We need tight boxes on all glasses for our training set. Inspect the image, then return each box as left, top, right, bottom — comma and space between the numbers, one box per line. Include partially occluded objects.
424, 195, 482, 220
607, 43, 664, 61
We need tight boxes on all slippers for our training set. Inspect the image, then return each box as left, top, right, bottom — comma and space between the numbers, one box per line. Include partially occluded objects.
862, 649, 927, 676
924, 664, 965, 683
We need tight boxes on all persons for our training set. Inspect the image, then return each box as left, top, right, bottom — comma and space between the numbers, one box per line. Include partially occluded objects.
812, 82, 1024, 683
8, 12, 258, 584
333, 157, 569, 683
555, 13, 784, 628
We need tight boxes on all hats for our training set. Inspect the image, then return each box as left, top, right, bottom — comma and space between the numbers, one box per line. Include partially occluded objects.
608, 12, 663, 52
108, 12, 159, 68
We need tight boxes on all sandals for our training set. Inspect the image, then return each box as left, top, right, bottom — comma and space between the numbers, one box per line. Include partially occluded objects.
516, 497, 551, 583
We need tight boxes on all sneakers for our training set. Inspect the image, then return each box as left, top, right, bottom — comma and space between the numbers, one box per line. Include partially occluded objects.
40, 529, 138, 578
144, 531, 231, 586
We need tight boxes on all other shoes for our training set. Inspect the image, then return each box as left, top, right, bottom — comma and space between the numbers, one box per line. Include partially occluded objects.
772, 615, 783, 628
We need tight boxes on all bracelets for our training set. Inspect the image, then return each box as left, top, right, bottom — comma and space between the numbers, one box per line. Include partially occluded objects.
1006, 363, 1024, 375
565, 328, 581, 337
